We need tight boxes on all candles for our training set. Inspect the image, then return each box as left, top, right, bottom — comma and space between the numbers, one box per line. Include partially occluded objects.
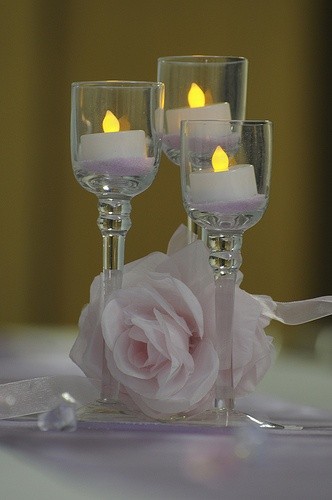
78, 82, 258, 204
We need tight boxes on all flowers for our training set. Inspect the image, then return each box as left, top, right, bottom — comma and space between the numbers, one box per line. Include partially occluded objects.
70, 223, 272, 420
